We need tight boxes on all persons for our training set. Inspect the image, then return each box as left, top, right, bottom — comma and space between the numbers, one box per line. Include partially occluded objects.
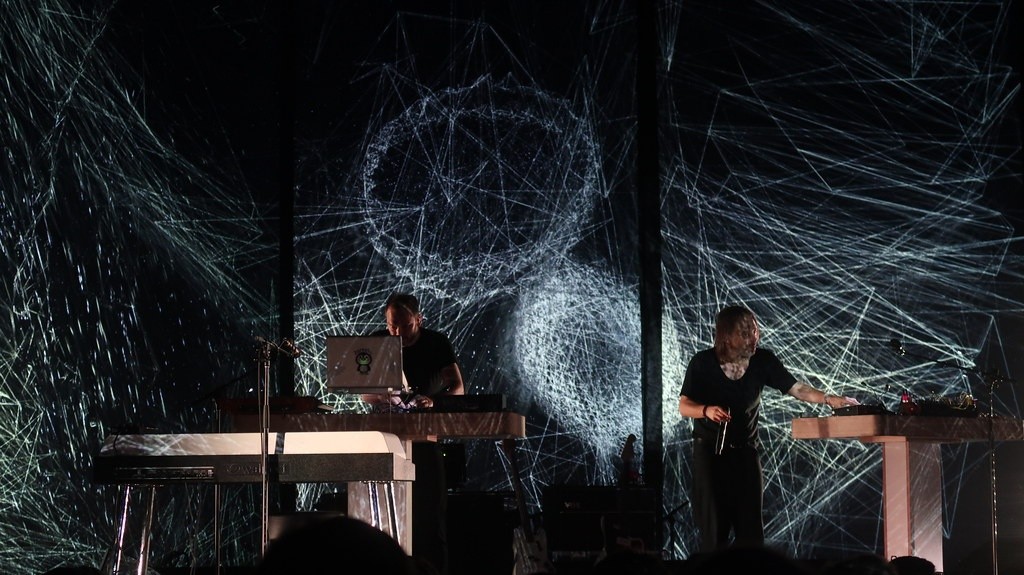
258, 517, 416, 575
364, 294, 463, 410
678, 305, 861, 554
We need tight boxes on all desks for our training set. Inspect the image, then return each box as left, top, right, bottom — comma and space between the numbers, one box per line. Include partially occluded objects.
243, 414, 526, 557
791, 414, 1024, 575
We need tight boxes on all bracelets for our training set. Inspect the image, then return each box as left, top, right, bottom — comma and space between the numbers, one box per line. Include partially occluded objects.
703, 405, 709, 418
824, 394, 829, 403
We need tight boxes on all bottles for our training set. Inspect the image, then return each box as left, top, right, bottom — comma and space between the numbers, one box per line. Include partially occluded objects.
930, 391, 936, 401
966, 397, 973, 405
901, 389, 911, 403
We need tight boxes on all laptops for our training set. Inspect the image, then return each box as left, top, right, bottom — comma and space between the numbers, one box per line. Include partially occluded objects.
326, 336, 414, 396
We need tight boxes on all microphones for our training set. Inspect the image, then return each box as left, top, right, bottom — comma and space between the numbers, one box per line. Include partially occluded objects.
715, 408, 731, 455
281, 338, 301, 358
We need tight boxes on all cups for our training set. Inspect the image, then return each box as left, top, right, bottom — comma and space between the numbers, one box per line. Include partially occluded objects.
972, 399, 977, 408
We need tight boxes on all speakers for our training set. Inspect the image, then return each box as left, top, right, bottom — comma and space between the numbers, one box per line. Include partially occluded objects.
559, 511, 658, 575
446, 489, 521, 575
